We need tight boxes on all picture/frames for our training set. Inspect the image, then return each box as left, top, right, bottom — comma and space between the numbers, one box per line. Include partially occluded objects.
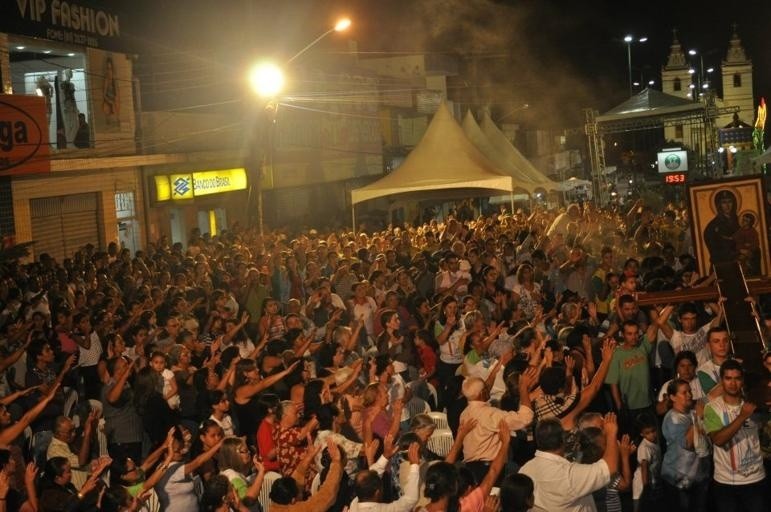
685, 175, 770, 288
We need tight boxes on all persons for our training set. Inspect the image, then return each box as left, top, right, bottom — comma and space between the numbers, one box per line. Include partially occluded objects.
36, 73, 53, 148
101, 57, 122, 130
2, 184, 771, 512
60, 68, 81, 118
74, 114, 91, 148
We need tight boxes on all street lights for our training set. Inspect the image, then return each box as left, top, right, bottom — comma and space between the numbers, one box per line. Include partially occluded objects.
621, 36, 716, 178
248, 18, 353, 238
495, 102, 530, 125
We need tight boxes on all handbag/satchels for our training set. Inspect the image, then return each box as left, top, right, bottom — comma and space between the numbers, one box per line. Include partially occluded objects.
691, 410, 713, 459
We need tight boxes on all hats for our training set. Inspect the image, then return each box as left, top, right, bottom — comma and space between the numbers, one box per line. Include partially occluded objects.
459, 259, 472, 271
